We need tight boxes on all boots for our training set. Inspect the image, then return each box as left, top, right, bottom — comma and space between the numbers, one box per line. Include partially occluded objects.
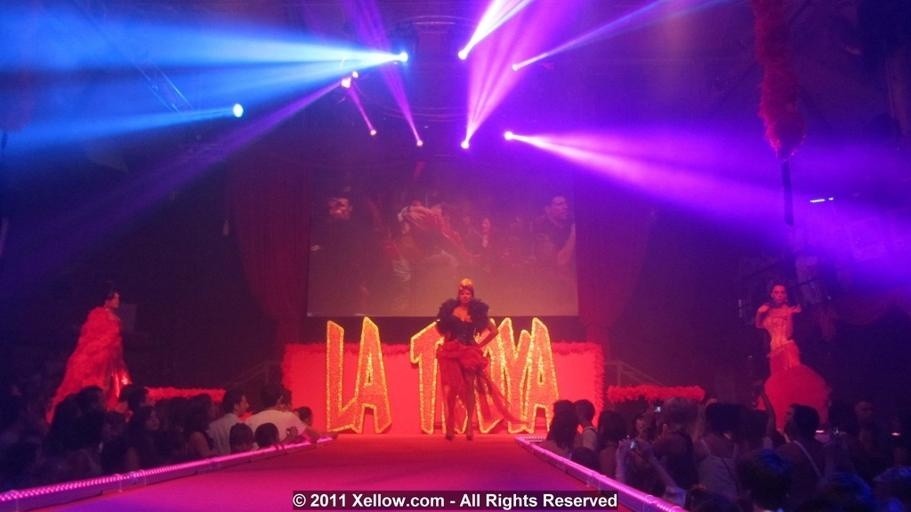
446, 416, 475, 440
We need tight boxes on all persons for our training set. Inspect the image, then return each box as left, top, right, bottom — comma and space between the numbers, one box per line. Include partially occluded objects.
45, 277, 131, 427
434, 277, 529, 440
0, 370, 320, 494
755, 282, 831, 439
306, 159, 579, 317
542, 391, 911, 512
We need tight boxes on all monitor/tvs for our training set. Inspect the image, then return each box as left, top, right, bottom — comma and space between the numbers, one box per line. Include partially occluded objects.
305, 165, 579, 320
